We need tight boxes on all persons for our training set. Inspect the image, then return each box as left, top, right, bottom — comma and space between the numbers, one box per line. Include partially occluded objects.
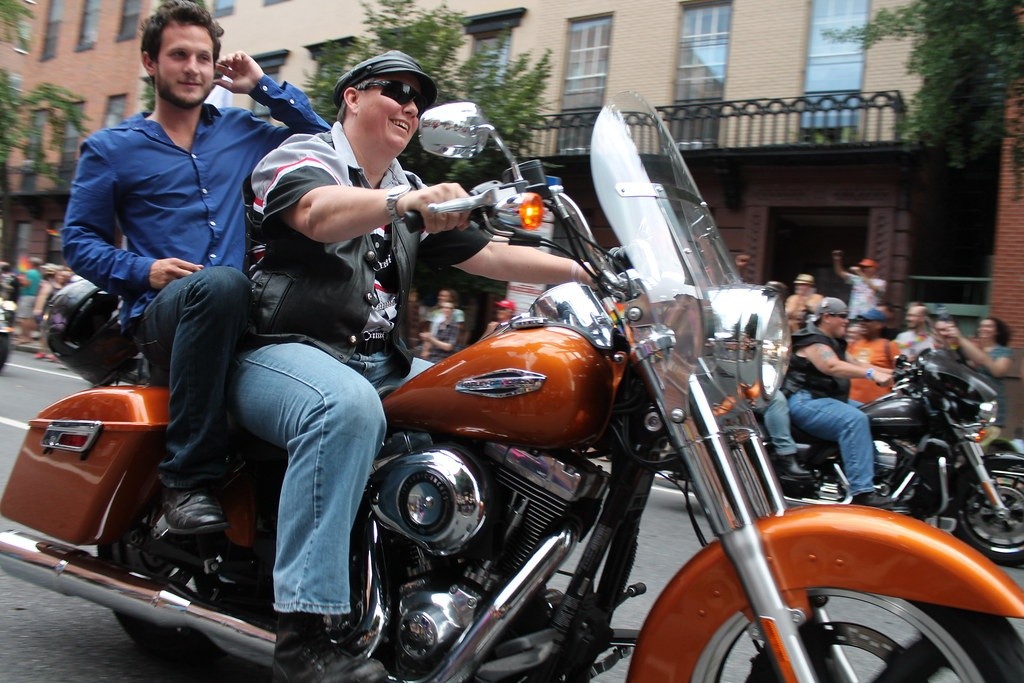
0, 260, 85, 379
739, 250, 1013, 510
406, 287, 515, 366
226, 51, 597, 683
60, 1, 332, 533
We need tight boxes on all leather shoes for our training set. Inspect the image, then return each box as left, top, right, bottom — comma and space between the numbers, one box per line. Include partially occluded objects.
162, 486, 231, 535
854, 491, 894, 508
777, 455, 813, 478
271, 613, 389, 683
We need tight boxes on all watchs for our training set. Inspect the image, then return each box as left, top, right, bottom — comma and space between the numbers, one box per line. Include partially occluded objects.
386, 185, 411, 223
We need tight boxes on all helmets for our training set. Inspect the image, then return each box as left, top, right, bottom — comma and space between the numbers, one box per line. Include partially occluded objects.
46, 282, 137, 384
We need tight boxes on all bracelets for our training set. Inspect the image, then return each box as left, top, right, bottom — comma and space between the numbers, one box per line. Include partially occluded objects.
571, 260, 584, 284
866, 369, 874, 379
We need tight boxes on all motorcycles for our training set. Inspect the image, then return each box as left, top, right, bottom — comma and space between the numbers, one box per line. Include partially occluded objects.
661, 315, 1024, 566
0, 272, 17, 371
0, 74, 1023, 683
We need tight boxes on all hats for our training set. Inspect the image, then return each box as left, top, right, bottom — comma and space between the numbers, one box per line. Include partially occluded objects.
333, 50, 438, 116
858, 259, 878, 267
856, 308, 886, 321
765, 281, 789, 292
793, 274, 815, 285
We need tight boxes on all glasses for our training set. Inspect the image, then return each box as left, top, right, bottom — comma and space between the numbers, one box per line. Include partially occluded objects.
830, 313, 848, 319
356, 78, 428, 119
